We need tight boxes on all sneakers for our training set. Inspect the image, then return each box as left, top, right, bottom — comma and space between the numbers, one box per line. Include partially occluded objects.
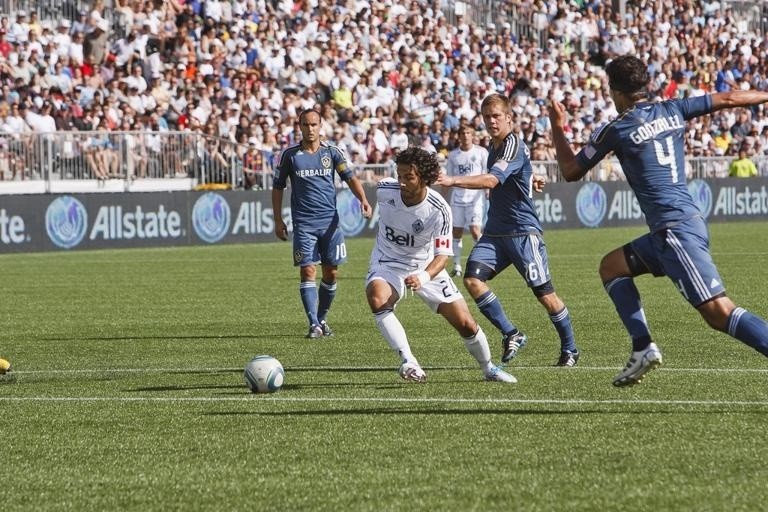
320, 321, 333, 336
557, 349, 580, 367
612, 342, 662, 388
307, 323, 323, 339
486, 364, 518, 383
399, 363, 426, 383
451, 270, 463, 277
502, 330, 527, 361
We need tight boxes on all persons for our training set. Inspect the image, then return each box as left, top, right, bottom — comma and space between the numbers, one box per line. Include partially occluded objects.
446, 125, 489, 278
437, 94, 581, 369
549, 55, 617, 158
549, 0, 768, 55
0, 0, 271, 190
685, 107, 768, 156
647, 55, 768, 100
548, 54, 768, 388
729, 150, 758, 177
366, 147, 518, 384
272, 109, 371, 338
271, 0, 548, 163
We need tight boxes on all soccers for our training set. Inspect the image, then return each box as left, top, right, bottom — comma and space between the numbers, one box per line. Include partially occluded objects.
244, 356, 285, 394
